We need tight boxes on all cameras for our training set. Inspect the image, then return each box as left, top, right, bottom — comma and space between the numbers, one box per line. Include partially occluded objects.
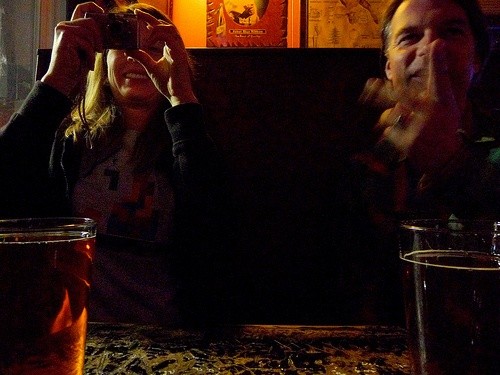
83, 11, 140, 50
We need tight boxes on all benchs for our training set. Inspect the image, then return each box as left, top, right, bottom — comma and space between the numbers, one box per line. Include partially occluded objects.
37, 49, 500, 324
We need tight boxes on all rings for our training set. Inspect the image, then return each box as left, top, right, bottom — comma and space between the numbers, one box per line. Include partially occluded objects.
393, 112, 408, 128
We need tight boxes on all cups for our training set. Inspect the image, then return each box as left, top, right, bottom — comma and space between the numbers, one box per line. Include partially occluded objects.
0, 218, 97, 375
400, 217, 500, 375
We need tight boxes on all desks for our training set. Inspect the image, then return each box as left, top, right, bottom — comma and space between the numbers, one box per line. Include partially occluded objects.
85, 323, 413, 372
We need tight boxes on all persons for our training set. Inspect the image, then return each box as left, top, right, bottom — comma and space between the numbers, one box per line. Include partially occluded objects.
0, 1, 243, 325
309, 0, 500, 323
